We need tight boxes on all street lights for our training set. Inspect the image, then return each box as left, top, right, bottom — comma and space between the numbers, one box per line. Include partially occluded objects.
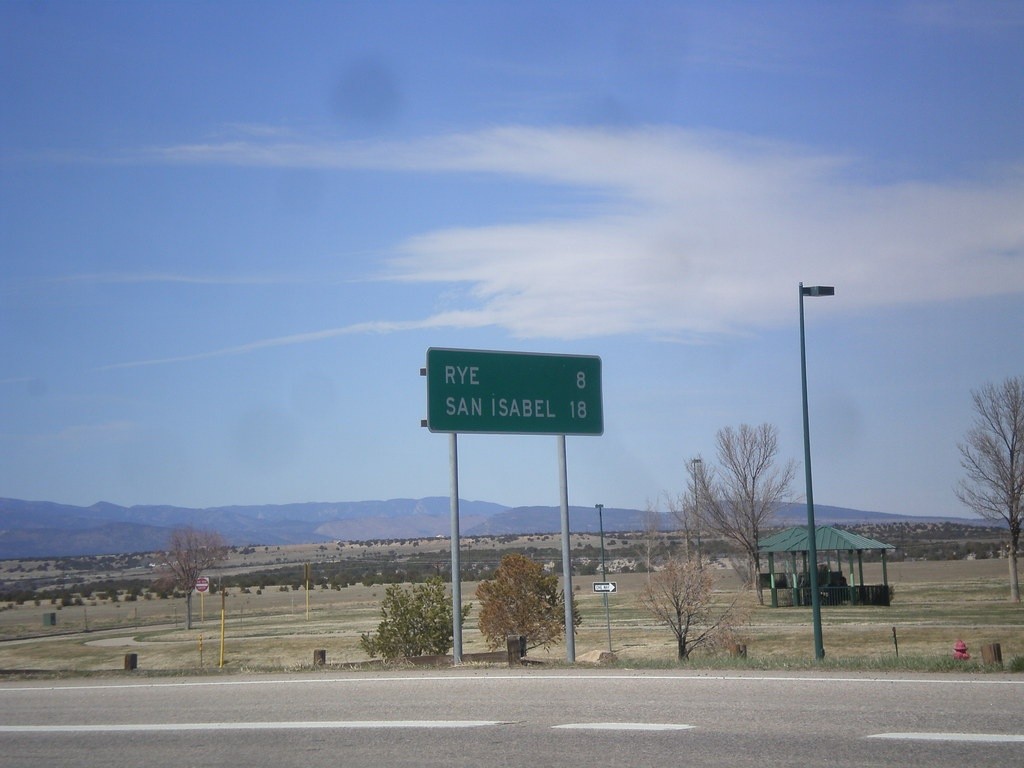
594, 503, 614, 651
799, 280, 837, 663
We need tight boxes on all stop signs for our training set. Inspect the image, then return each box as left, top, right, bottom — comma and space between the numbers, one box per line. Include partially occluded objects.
194, 577, 209, 593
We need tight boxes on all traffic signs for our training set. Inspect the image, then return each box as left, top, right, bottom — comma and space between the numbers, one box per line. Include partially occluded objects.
592, 582, 618, 594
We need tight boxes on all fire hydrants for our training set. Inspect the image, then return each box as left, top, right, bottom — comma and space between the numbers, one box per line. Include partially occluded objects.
954, 638, 970, 661
